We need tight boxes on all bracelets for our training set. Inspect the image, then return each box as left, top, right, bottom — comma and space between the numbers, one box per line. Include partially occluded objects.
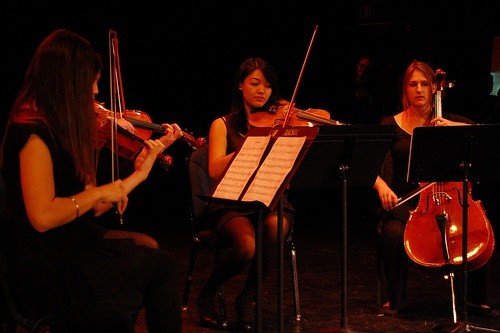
69, 196, 80, 218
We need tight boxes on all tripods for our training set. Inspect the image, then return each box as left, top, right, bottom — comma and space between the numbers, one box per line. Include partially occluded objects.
410, 123, 500, 333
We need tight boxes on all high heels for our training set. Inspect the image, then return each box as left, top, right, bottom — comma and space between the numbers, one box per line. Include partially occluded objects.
234, 294, 255, 333
196, 296, 221, 328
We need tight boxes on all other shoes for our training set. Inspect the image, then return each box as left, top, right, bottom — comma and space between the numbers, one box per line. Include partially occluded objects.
384, 288, 399, 309
462, 300, 500, 317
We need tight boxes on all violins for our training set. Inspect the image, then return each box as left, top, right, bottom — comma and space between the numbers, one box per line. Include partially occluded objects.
248, 99, 349, 127
93, 100, 206, 165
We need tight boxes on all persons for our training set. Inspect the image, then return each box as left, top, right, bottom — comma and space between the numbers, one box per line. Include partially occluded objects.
363, 60, 495, 313
193, 58, 294, 333
0, 29, 184, 333
340, 52, 379, 122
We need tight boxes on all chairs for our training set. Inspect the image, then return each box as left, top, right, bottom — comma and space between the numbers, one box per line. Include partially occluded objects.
182, 145, 303, 323
375, 204, 466, 311
0, 244, 139, 333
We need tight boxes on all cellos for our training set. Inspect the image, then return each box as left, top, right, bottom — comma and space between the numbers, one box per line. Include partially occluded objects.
403, 68, 495, 324
